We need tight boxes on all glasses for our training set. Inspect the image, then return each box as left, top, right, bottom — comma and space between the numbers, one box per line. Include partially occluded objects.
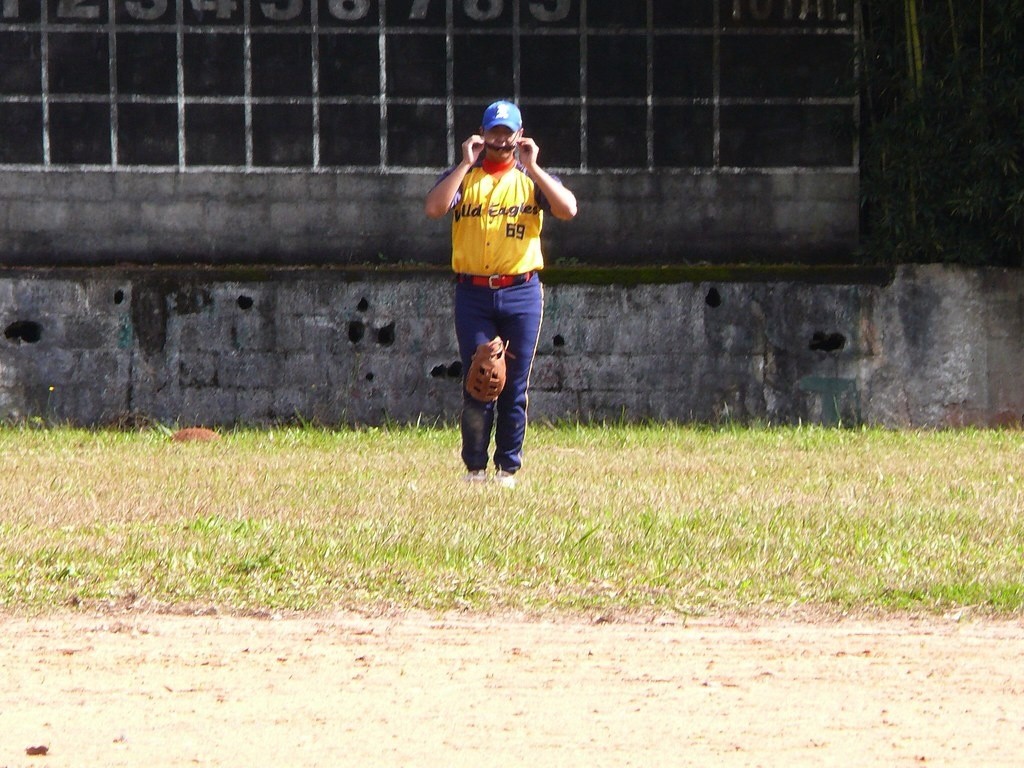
482, 128, 521, 151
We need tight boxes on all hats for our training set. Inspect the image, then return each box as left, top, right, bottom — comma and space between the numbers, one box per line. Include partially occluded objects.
482, 100, 522, 132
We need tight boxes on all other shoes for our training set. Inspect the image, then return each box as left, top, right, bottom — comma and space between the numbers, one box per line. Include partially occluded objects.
464, 469, 487, 480
493, 469, 516, 490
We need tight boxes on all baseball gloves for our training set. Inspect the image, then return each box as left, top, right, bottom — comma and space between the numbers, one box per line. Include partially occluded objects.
465, 335, 516, 402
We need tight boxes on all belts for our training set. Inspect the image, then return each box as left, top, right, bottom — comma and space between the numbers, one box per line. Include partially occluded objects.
456, 271, 533, 289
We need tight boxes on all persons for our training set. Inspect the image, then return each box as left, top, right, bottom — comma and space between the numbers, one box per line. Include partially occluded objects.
423, 101, 578, 491
